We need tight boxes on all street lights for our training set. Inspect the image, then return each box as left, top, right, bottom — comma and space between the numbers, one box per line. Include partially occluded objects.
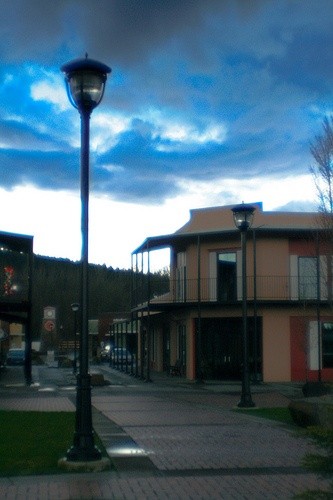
69, 302, 83, 383
231, 201, 258, 407
57, 53, 117, 471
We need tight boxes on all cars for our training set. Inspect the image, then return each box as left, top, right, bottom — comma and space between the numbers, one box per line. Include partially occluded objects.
110, 347, 134, 364
